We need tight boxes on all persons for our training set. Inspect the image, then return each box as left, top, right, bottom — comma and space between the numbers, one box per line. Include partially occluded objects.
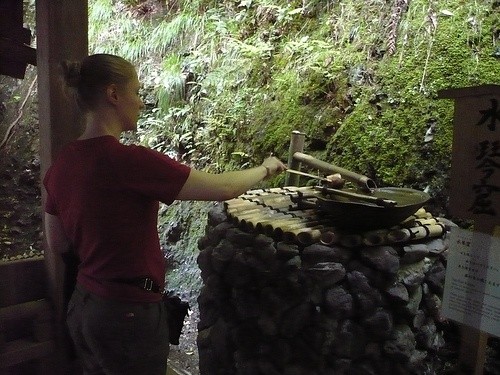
42, 54, 290, 375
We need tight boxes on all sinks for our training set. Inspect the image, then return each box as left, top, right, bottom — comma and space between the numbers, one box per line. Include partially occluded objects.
304, 186, 432, 227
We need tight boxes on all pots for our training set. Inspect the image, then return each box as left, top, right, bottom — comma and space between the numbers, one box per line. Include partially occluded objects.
314, 188, 432, 226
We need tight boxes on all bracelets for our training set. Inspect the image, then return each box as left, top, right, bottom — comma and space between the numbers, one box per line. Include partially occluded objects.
261, 164, 271, 180
